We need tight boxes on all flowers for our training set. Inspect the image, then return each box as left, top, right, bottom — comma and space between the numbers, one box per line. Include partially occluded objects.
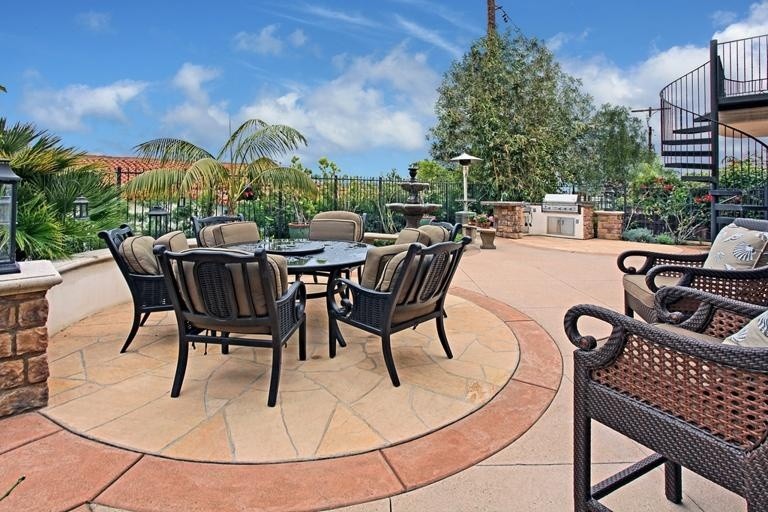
472, 213, 494, 228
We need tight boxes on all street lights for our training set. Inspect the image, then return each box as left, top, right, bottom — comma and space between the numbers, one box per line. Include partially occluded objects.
451, 154, 483, 211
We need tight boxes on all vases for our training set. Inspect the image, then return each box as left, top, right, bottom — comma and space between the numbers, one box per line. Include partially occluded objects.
477, 228, 496, 249
288, 222, 310, 239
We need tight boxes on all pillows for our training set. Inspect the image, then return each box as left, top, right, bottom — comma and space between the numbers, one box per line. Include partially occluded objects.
118, 236, 158, 274
309, 218, 356, 241
212, 219, 260, 246
171, 247, 281, 317
703, 223, 768, 269
199, 223, 226, 247
152, 229, 189, 274
394, 227, 430, 247
361, 242, 428, 294
267, 253, 288, 295
720, 310, 767, 347
418, 224, 450, 247
313, 211, 363, 241
376, 253, 438, 325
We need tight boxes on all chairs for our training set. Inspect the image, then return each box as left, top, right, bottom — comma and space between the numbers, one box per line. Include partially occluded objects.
563, 286, 768, 512
326, 234, 471, 385
409, 221, 462, 330
295, 210, 367, 303
191, 213, 245, 247
154, 241, 308, 407
617, 218, 768, 323
98, 223, 207, 354
